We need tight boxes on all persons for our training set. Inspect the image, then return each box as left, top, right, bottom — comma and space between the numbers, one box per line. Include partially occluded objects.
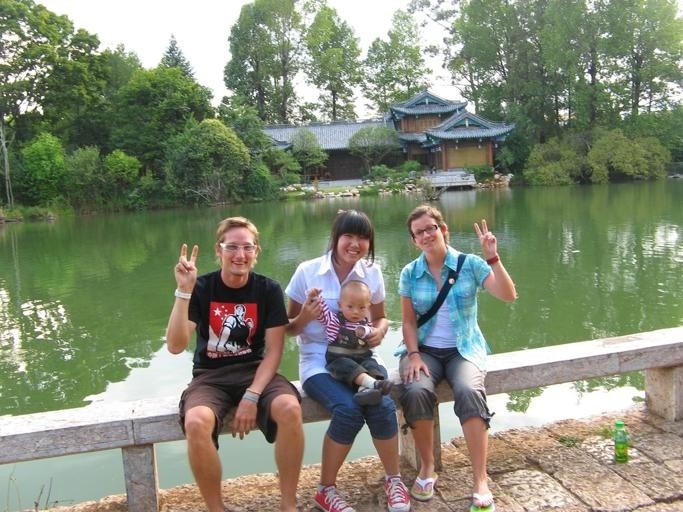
395, 200, 526, 512
162, 213, 307, 512
304, 277, 398, 411
283, 210, 418, 512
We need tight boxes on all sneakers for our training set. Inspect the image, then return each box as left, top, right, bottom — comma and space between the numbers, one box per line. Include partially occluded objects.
383, 476, 411, 512
353, 388, 382, 405
374, 379, 395, 396
313, 485, 356, 511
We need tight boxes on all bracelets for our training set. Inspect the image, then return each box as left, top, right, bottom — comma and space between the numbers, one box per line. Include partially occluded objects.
245, 388, 262, 396
241, 392, 259, 404
173, 289, 194, 303
482, 254, 500, 267
408, 351, 420, 358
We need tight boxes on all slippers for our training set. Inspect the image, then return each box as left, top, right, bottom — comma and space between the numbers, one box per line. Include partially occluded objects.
470, 493, 494, 511
411, 472, 439, 501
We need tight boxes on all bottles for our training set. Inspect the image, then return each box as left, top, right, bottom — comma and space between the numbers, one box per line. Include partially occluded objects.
613, 421, 628, 463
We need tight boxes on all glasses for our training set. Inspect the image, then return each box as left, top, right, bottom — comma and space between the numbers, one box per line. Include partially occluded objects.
414, 224, 441, 236
219, 242, 258, 252
